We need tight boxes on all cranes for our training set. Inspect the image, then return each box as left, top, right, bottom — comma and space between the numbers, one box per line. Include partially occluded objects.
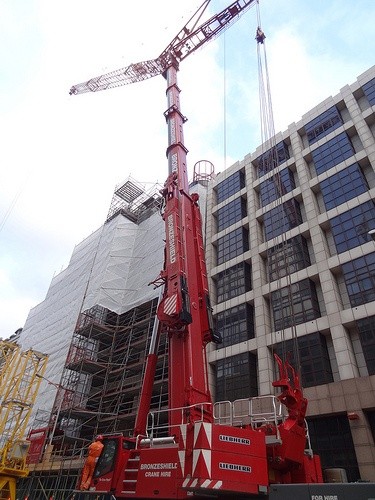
70, 0, 277, 425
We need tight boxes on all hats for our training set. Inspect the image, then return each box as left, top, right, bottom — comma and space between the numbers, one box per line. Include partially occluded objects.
96, 435, 103, 440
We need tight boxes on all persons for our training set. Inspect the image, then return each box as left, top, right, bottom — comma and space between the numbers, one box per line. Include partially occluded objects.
79, 434, 105, 491
